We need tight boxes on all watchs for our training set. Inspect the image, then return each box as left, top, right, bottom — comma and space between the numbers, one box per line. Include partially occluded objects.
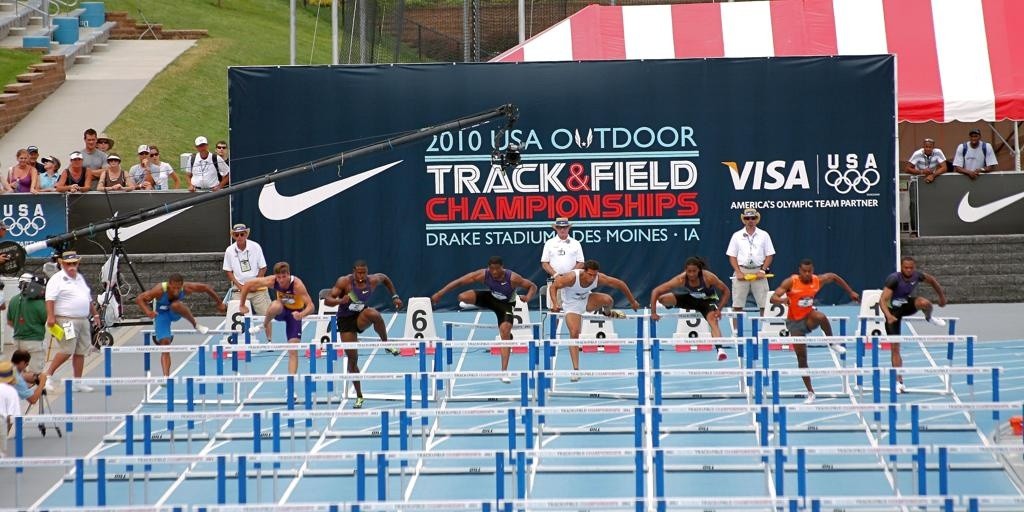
981, 167, 986, 173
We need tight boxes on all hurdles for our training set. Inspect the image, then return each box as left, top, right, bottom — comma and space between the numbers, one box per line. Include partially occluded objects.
499, 310, 1018, 509
2, 314, 544, 511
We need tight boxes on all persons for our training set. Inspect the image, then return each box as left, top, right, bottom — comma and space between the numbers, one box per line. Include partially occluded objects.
650, 256, 730, 361
879, 257, 945, 395
135, 273, 225, 385
549, 259, 640, 382
324, 260, 403, 409
238, 262, 315, 399
0, 249, 100, 459
770, 259, 860, 406
0, 220, 9, 264
952, 128, 998, 179
541, 218, 584, 327
222, 224, 274, 353
905, 138, 947, 184
431, 256, 537, 383
725, 208, 775, 334
0, 130, 231, 192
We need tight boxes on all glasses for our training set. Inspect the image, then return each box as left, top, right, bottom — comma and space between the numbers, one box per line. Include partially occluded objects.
235, 233, 244, 237
743, 217, 757, 220
217, 146, 226, 149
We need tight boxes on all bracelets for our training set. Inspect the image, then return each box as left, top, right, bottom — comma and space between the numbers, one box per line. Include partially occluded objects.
931, 173, 936, 178
391, 295, 399, 300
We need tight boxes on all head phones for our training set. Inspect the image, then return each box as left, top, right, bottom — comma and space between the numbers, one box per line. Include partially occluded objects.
923, 152, 934, 159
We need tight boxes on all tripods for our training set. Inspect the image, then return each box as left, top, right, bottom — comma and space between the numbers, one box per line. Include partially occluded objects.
24, 388, 62, 438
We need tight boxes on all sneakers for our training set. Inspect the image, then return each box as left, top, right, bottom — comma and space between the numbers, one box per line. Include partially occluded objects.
896, 381, 905, 393
161, 376, 168, 388
353, 397, 365, 408
267, 342, 274, 353
608, 310, 627, 319
72, 383, 94, 392
45, 375, 53, 391
385, 347, 401, 356
928, 317, 944, 326
803, 392, 816, 404
286, 389, 297, 400
459, 301, 476, 307
197, 325, 209, 334
570, 376, 582, 382
717, 348, 728, 361
502, 377, 511, 383
831, 343, 846, 354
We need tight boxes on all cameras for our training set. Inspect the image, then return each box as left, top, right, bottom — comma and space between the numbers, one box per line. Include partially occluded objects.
33, 374, 41, 384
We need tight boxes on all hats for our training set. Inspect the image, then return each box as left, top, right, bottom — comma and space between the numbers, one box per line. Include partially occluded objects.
26, 133, 207, 163
0, 360, 15, 384
58, 251, 81, 265
741, 209, 760, 224
18, 273, 34, 283
552, 218, 572, 231
230, 224, 250, 240
969, 128, 981, 136
